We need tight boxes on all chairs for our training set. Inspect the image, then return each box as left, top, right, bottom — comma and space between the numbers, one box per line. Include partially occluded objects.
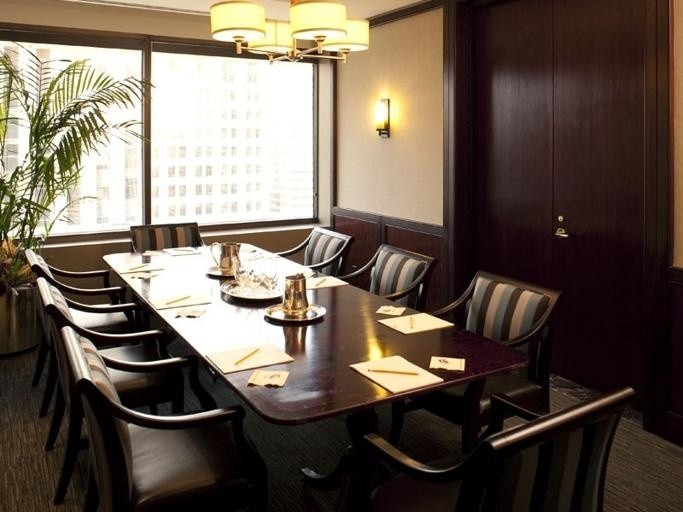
276, 227, 354, 268
301, 387, 636, 512
36, 278, 165, 451
60, 326, 246, 512
131, 222, 204, 251
391, 271, 562, 455
335, 244, 437, 300
24, 249, 125, 414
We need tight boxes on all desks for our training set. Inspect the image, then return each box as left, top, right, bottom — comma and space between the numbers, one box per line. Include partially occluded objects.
103, 243, 532, 449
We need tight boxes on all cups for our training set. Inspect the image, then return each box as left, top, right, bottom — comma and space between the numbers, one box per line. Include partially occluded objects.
266, 256, 280, 296
249, 257, 265, 295
232, 255, 250, 295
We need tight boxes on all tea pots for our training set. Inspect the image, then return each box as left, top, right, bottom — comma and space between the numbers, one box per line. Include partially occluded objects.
280, 273, 311, 319
210, 243, 243, 275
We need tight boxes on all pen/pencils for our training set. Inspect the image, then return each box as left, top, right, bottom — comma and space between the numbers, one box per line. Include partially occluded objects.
410, 316, 413, 328
166, 296, 190, 305
368, 369, 418, 375
234, 348, 259, 365
172, 247, 193, 252
128, 264, 147, 270
315, 278, 326, 286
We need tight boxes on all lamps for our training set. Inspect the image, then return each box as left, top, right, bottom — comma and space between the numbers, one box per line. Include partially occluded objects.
210, 0, 369, 64
375, 99, 390, 139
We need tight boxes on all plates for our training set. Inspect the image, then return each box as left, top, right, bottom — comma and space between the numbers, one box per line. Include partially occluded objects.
218, 279, 286, 300
205, 264, 248, 279
261, 302, 326, 322
